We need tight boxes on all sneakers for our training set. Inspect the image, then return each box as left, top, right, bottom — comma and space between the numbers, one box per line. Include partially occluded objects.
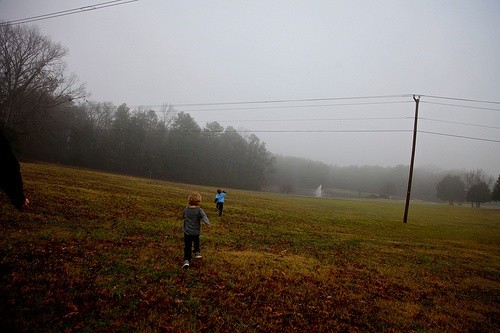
195, 252, 202, 258
181, 259, 189, 269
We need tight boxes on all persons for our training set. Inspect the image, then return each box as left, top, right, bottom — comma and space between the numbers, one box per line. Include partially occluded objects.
0, 131, 30, 211
214, 189, 227, 216
181, 193, 211, 269
